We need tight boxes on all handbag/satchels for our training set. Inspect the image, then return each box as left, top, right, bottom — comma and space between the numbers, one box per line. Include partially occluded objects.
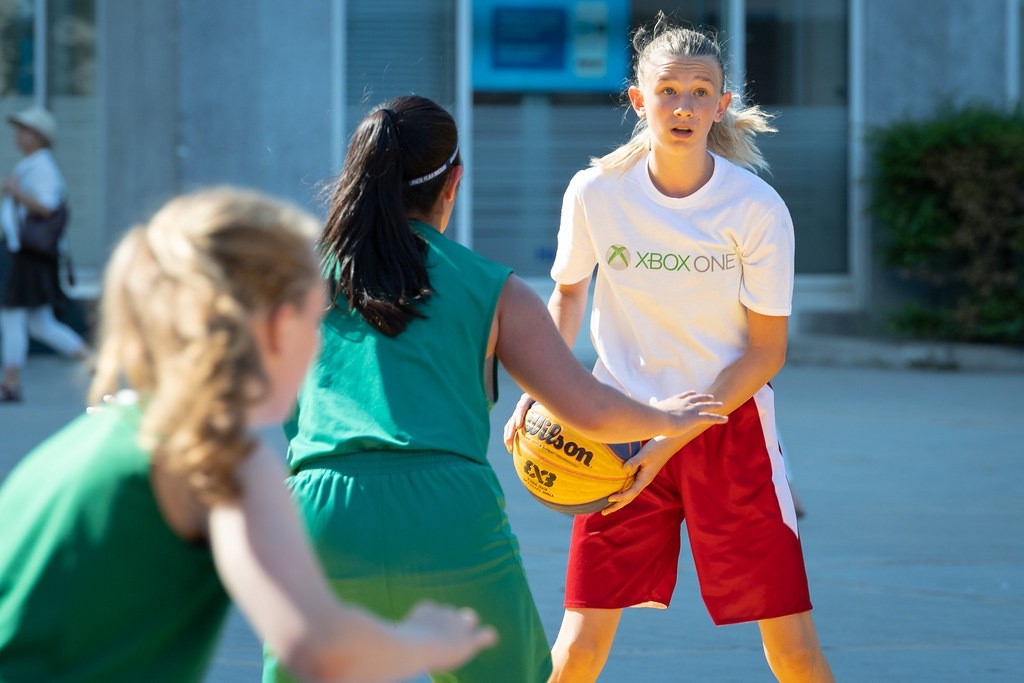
20, 207, 67, 254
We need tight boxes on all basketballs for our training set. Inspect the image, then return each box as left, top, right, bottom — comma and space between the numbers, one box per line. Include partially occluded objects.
512, 397, 644, 518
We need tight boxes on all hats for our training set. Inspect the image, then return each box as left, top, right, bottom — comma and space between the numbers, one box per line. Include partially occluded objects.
7, 103, 56, 145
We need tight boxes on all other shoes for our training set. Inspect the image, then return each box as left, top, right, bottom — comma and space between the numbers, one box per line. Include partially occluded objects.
0, 384, 23, 402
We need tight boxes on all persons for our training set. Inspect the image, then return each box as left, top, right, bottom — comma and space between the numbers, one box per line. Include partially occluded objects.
261, 95, 728, 683
0, 187, 497, 683
504, 28, 839, 683
0, 105, 96, 402
